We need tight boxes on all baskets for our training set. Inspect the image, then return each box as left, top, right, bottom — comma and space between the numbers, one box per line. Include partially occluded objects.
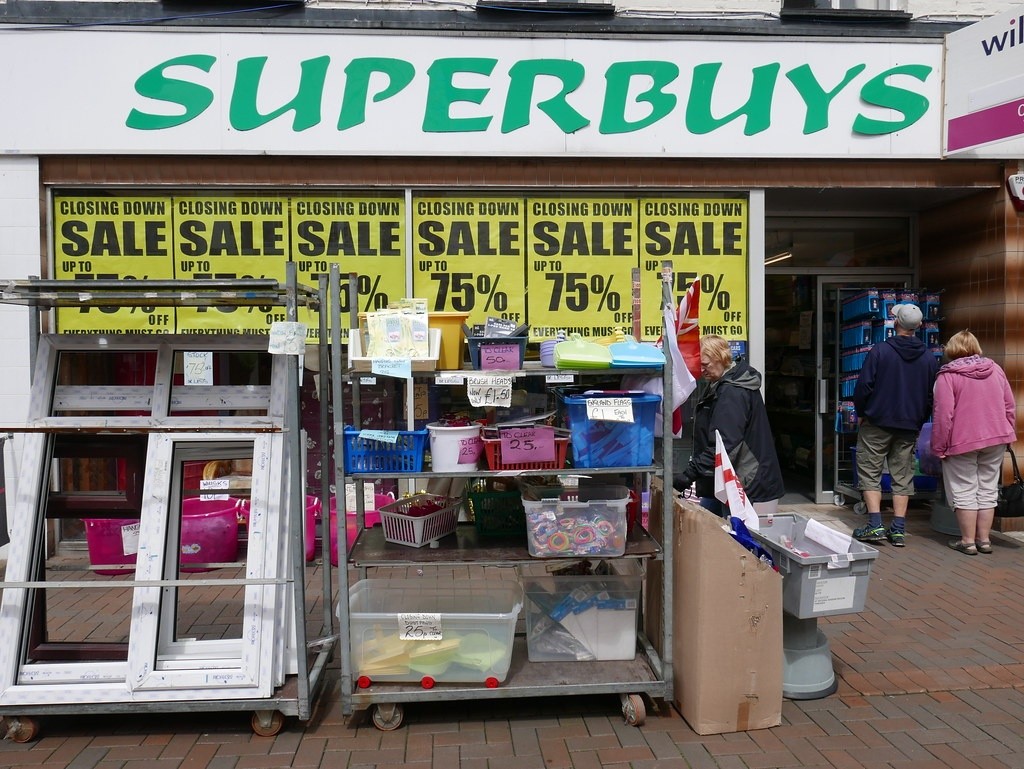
379, 493, 464, 548
480, 433, 569, 471
345, 427, 429, 473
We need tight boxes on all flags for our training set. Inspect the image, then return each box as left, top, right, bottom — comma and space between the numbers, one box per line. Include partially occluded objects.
670, 276, 702, 441
714, 429, 760, 531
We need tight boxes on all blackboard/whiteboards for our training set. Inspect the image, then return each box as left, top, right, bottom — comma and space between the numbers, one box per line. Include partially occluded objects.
183, 351, 213, 386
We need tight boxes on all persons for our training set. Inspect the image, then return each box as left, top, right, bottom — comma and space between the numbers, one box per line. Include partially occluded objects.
853, 304, 939, 546
933, 329, 1018, 555
672, 334, 785, 518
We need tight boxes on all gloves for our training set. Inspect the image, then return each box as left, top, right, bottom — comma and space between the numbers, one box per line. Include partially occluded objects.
673, 474, 693, 492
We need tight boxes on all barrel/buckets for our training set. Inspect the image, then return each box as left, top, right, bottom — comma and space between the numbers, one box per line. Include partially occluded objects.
79, 518, 139, 575
180, 496, 241, 573
320, 491, 395, 567
240, 494, 320, 562
425, 421, 482, 473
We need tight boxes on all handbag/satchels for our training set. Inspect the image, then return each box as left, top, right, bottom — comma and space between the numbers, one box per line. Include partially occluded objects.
993, 447, 1024, 518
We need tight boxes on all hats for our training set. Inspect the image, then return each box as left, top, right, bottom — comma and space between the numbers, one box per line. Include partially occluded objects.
891, 303, 923, 330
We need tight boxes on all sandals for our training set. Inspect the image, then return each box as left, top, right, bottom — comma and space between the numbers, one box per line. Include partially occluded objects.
975, 542, 993, 553
947, 539, 978, 555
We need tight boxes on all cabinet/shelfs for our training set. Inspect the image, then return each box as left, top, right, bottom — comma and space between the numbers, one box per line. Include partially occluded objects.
765, 306, 834, 416
300, 378, 395, 499
326, 261, 674, 727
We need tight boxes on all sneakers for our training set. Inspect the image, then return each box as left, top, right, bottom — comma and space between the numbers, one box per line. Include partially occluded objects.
852, 522, 887, 541
885, 533, 906, 546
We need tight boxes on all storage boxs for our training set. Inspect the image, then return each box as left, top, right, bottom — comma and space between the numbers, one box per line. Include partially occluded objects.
465, 335, 529, 371
357, 311, 473, 370
518, 483, 633, 558
552, 385, 662, 467
644, 473, 786, 734
517, 561, 647, 661
747, 512, 880, 621
334, 574, 524, 686
